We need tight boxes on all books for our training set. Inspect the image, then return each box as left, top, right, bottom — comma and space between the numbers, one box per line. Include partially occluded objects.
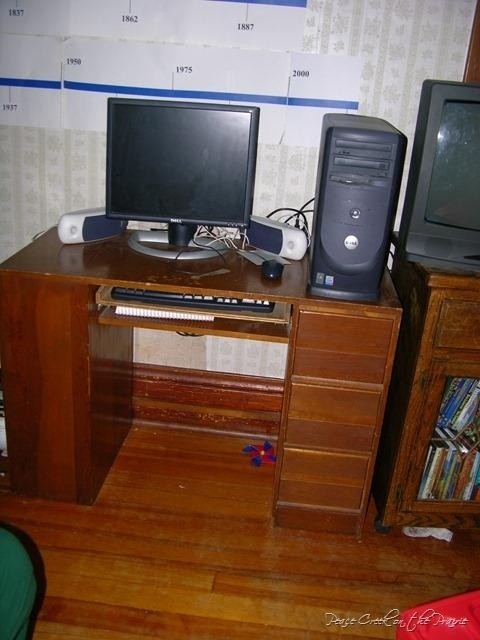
416, 376, 480, 502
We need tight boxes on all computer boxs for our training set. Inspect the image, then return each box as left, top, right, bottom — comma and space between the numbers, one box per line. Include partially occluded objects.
307, 113, 408, 305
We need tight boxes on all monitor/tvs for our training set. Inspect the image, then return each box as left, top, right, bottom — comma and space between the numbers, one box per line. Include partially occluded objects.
106, 97, 260, 260
398, 78, 479, 272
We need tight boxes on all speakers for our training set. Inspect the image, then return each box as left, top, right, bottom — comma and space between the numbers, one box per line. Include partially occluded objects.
248, 215, 308, 261
57, 208, 128, 244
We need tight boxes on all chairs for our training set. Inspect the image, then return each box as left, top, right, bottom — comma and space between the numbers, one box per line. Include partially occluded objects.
1, 521, 49, 639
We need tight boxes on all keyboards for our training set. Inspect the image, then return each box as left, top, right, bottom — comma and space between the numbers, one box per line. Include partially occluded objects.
111, 286, 275, 313
261, 260, 284, 287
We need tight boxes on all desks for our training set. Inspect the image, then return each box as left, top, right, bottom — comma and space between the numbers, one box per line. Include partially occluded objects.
0, 224, 403, 542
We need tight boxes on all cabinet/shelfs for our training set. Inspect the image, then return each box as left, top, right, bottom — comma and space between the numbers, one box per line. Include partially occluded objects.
370, 243, 480, 540
